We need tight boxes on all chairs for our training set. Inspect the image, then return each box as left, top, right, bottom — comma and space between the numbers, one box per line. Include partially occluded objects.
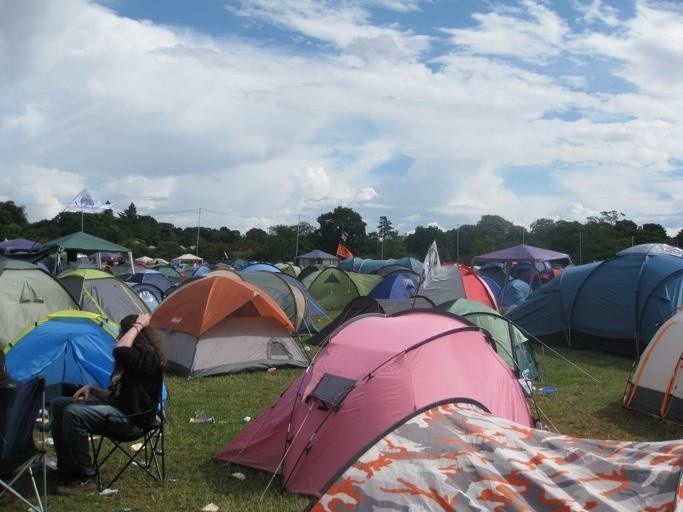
0, 376, 165, 511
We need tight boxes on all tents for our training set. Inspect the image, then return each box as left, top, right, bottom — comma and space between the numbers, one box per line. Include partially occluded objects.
2, 230, 683, 355
2, 309, 170, 426
301, 395, 682, 512
140, 274, 311, 377
616, 309, 683, 428
211, 308, 534, 499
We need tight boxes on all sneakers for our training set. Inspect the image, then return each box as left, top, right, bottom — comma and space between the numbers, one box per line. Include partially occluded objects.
48, 470, 91, 495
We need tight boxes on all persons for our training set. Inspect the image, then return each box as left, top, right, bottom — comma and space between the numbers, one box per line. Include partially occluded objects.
44, 314, 165, 496
